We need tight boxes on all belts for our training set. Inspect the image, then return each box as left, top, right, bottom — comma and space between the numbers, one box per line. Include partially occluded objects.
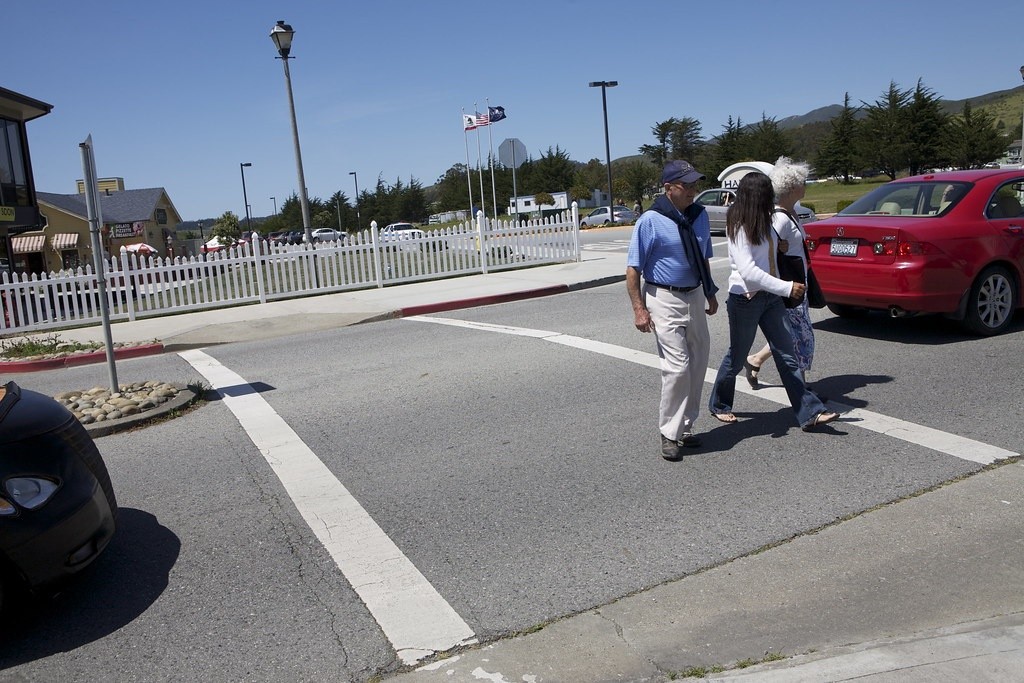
645, 280, 701, 292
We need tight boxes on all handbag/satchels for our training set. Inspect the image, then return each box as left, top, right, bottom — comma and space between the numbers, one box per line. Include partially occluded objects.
771, 226, 806, 308
807, 266, 826, 308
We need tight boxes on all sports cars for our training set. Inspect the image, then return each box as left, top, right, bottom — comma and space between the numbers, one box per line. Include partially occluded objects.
0, 380, 118, 601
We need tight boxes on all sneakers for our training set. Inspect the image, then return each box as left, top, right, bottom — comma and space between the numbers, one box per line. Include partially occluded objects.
660, 433, 680, 458
677, 434, 703, 446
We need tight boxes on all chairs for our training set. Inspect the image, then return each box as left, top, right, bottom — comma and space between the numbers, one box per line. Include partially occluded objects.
937, 201, 951, 214
993, 196, 1024, 219
880, 202, 902, 216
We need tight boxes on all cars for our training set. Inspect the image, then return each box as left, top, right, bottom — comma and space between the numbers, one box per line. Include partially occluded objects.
695, 188, 820, 238
580, 204, 635, 229
380, 222, 425, 241
801, 167, 1024, 332
239, 228, 305, 246
302, 226, 350, 244
986, 162, 1000, 169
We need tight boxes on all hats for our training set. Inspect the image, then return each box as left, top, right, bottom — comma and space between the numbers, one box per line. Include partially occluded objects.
662, 160, 706, 184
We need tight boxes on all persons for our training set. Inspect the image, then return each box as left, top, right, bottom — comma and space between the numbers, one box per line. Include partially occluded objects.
744, 159, 829, 402
709, 172, 840, 432
627, 161, 719, 460
133, 251, 158, 264
618, 199, 627, 206
261, 237, 270, 250
633, 200, 643, 214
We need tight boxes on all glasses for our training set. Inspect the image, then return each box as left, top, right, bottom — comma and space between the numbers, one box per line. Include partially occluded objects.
669, 179, 697, 188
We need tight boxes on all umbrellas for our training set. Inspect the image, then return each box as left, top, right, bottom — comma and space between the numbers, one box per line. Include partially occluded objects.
126, 243, 158, 253
201, 235, 245, 252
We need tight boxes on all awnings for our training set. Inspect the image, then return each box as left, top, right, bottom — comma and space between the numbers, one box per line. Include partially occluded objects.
10, 235, 46, 253
163, 228, 177, 240
53, 233, 79, 249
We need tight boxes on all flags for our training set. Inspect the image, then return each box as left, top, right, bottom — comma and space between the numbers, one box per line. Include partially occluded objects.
488, 106, 506, 122
464, 115, 476, 130
476, 111, 492, 126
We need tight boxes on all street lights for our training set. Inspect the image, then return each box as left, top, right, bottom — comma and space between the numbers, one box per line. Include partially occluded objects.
197, 220, 204, 240
588, 79, 618, 225
349, 171, 361, 232
267, 19, 317, 251
247, 204, 254, 231
167, 235, 176, 265
238, 162, 254, 244
270, 197, 277, 217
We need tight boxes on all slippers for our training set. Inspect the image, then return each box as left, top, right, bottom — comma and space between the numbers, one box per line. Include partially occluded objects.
709, 407, 736, 421
744, 357, 760, 389
802, 411, 840, 429
